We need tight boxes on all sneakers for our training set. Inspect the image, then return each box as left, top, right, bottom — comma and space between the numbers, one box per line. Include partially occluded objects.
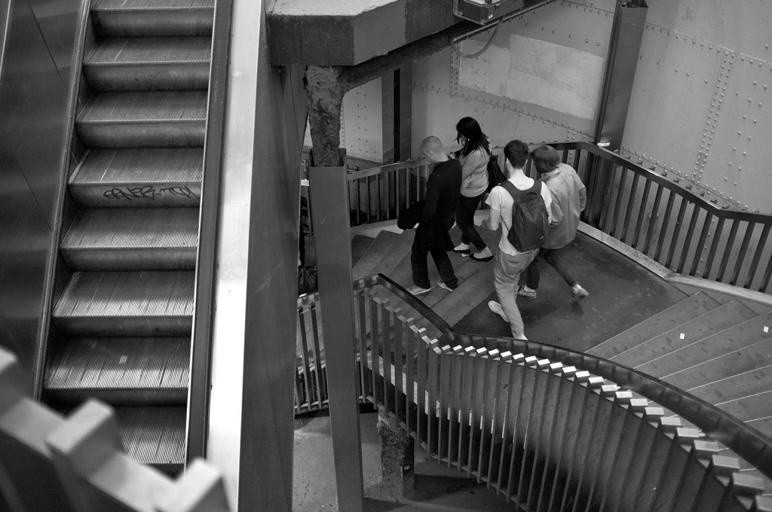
487, 299, 509, 322
405, 284, 433, 296
437, 279, 455, 292
517, 285, 536, 298
568, 288, 589, 304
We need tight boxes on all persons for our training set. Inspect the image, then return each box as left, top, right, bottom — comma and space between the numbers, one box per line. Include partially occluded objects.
451, 117, 496, 261
512, 145, 590, 299
407, 136, 459, 296
484, 140, 551, 343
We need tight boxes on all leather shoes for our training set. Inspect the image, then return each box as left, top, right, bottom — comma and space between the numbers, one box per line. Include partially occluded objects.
452, 244, 470, 254
471, 248, 494, 262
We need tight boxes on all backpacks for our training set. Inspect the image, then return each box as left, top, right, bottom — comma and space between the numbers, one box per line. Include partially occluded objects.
497, 177, 549, 253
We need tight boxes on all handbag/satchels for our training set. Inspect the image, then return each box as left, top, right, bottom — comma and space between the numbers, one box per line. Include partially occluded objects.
488, 155, 505, 192
